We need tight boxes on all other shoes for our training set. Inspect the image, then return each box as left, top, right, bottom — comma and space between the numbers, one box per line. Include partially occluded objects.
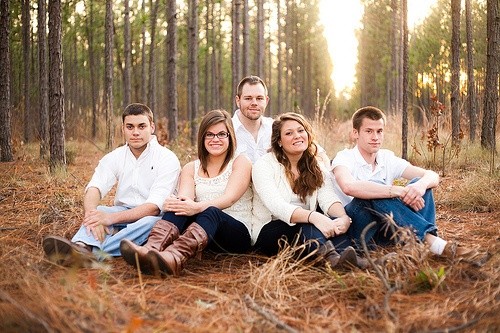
442, 242, 489, 263
43, 235, 91, 264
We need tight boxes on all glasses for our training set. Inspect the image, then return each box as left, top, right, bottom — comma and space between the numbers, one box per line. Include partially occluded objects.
204, 132, 230, 139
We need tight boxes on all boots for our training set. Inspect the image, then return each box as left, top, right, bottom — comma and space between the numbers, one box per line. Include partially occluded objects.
147, 221, 209, 276
119, 220, 180, 274
358, 251, 398, 270
317, 240, 357, 269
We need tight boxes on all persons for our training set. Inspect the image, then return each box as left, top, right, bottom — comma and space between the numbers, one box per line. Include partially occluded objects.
332, 106, 456, 258
120, 109, 252, 277
42, 103, 181, 268
251, 112, 399, 270
231, 75, 276, 165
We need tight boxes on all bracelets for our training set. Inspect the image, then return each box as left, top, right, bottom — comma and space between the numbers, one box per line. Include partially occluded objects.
341, 214, 352, 226
307, 210, 315, 223
389, 186, 393, 199
